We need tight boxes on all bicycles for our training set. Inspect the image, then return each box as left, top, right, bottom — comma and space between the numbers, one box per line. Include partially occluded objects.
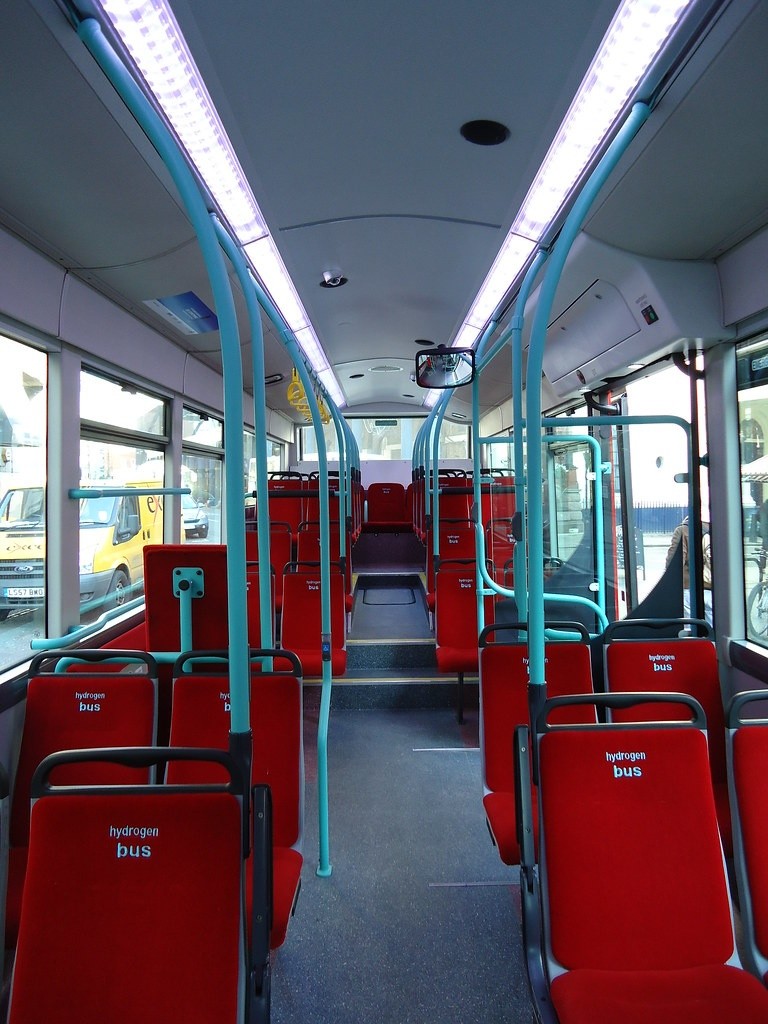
747, 550, 768, 637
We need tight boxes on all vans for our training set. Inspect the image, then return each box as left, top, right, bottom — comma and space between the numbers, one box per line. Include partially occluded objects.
0, 479, 187, 623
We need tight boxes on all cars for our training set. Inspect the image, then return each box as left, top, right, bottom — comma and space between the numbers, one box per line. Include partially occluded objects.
181, 494, 208, 538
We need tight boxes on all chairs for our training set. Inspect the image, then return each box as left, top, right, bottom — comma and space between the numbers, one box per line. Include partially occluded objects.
5, 649, 159, 948
272, 560, 347, 676
242, 520, 291, 612
480, 620, 600, 866
601, 617, 733, 859
365, 482, 411, 536
514, 692, 768, 1024
725, 688, 768, 986
405, 468, 564, 602
5, 745, 271, 1024
246, 561, 276, 674
163, 650, 303, 951
296, 520, 354, 612
434, 558, 498, 724
256, 471, 364, 550
